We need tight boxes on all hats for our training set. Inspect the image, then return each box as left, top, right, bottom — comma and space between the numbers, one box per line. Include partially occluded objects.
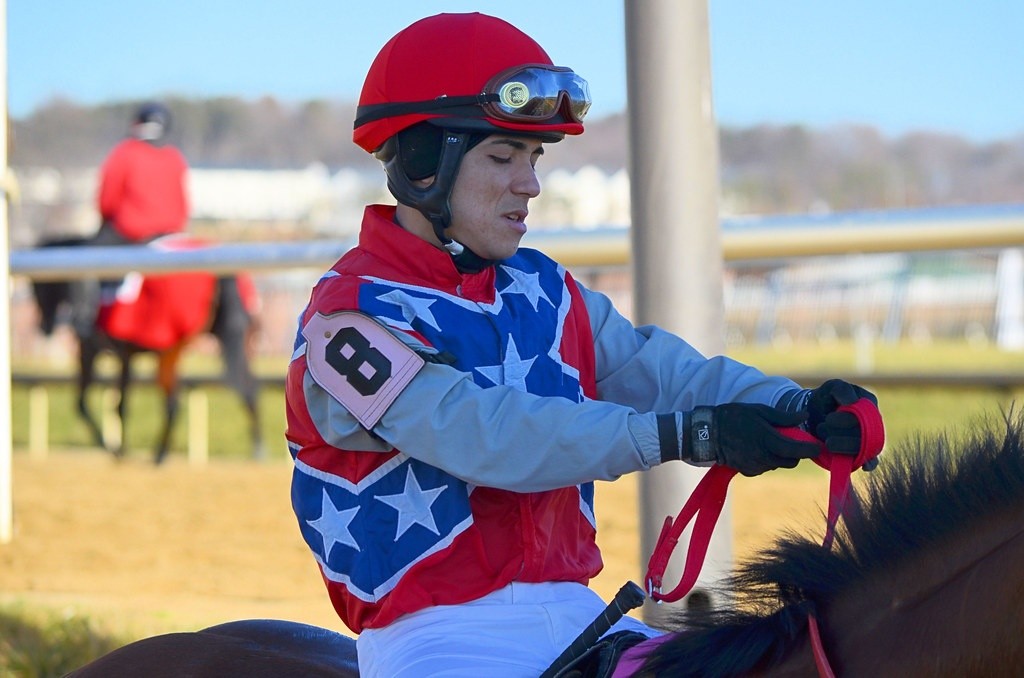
137, 103, 173, 133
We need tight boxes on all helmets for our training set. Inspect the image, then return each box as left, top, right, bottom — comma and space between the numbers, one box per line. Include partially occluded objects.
352, 12, 585, 153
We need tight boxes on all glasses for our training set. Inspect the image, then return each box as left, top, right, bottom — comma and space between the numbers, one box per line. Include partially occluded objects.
480, 63, 593, 123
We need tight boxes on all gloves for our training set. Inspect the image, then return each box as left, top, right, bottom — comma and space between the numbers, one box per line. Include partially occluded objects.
805, 379, 879, 455
682, 403, 820, 478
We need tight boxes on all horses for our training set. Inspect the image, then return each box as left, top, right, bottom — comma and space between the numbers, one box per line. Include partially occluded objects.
58, 397, 1024, 678
28, 232, 265, 465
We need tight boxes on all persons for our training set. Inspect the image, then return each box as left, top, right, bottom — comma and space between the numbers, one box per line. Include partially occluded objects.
282, 9, 887, 678
67, 103, 194, 342
